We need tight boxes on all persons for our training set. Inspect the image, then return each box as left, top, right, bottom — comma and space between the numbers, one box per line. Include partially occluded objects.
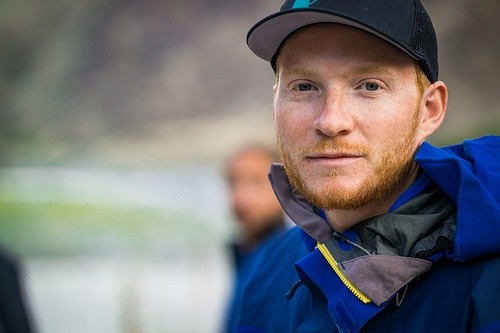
218, 144, 290, 277
223, 0, 500, 332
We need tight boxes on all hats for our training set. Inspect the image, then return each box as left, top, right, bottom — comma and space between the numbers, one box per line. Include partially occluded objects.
247, 0, 440, 85
